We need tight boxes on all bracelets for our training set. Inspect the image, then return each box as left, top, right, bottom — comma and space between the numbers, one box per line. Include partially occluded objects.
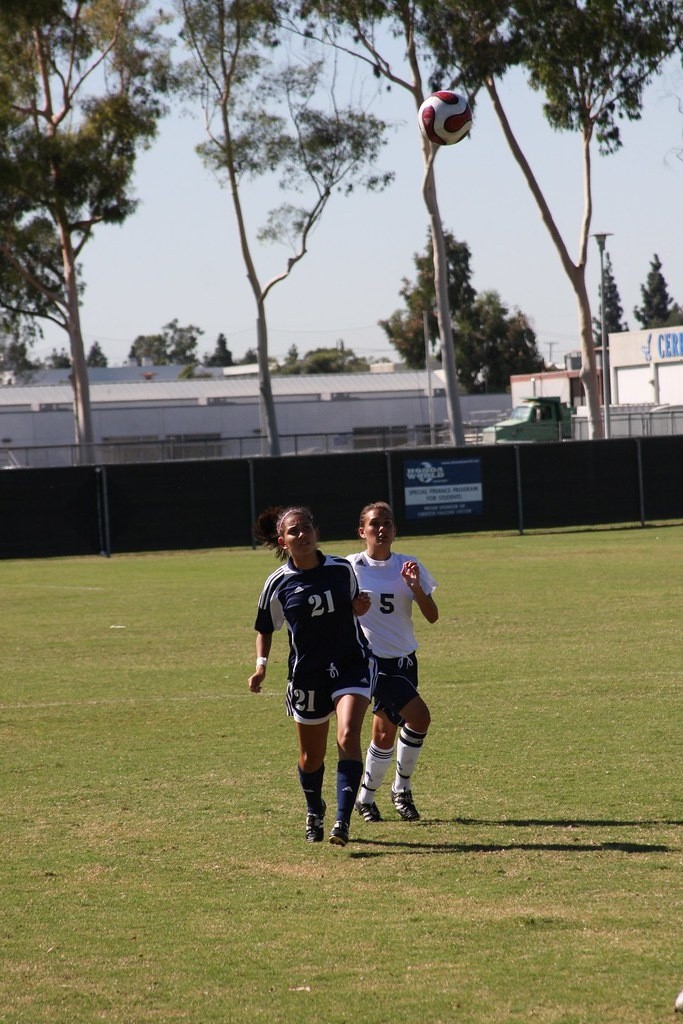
255, 657, 268, 670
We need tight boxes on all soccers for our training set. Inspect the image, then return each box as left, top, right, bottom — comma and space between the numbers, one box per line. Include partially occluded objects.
417, 90, 473, 146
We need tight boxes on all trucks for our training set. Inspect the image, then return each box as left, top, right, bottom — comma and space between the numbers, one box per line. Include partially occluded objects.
480, 395, 671, 446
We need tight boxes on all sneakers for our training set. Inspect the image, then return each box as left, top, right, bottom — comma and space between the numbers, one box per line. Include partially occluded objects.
305, 799, 326, 842
390, 781, 420, 821
328, 820, 350, 846
354, 800, 383, 823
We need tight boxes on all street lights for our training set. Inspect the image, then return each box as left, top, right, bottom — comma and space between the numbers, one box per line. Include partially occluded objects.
589, 227, 616, 440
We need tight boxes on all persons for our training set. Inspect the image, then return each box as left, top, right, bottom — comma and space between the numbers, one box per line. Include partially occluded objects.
344, 502, 438, 822
247, 506, 378, 846
